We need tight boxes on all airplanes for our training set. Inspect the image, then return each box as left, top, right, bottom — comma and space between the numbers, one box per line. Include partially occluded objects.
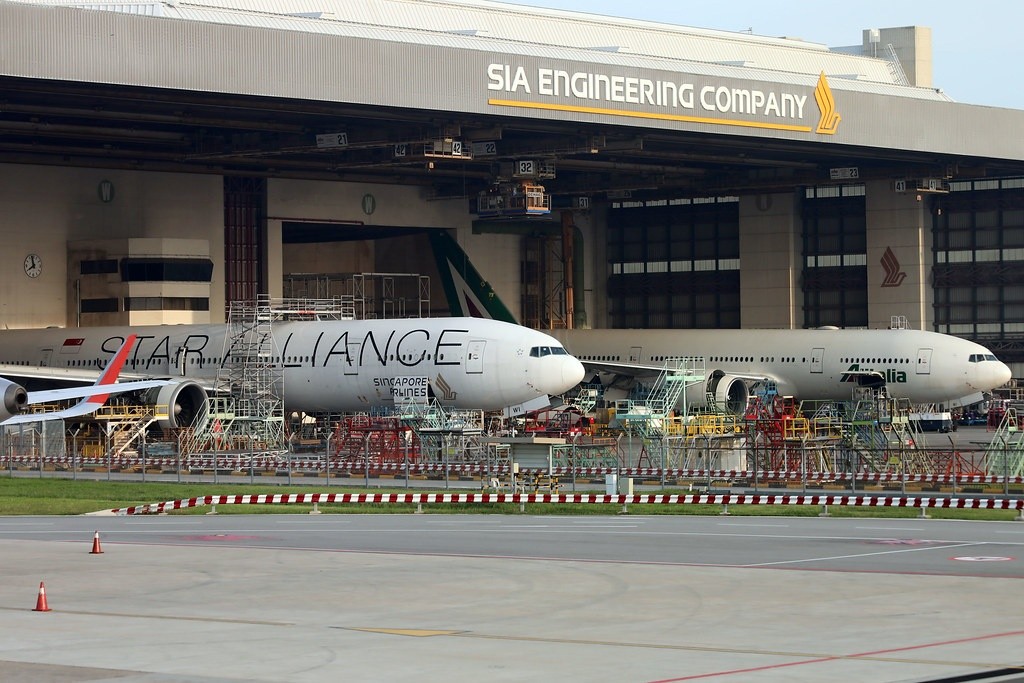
527, 329, 1011, 426
0, 316, 585, 439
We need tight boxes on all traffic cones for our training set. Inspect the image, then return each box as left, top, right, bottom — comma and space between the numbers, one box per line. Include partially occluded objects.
88, 530, 103, 553
31, 582, 52, 612
